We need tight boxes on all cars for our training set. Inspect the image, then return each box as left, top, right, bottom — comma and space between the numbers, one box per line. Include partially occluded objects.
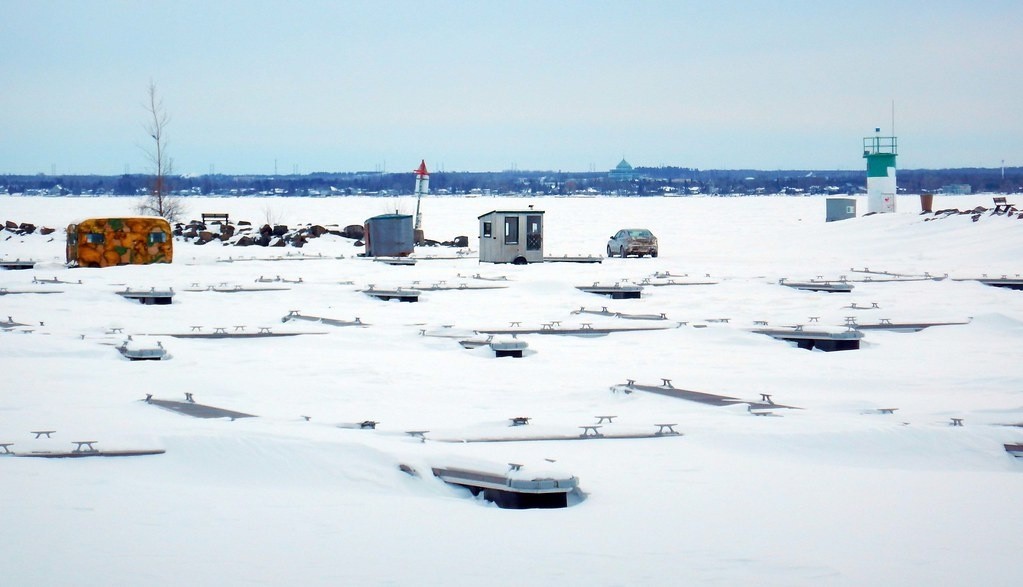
605, 228, 659, 258
